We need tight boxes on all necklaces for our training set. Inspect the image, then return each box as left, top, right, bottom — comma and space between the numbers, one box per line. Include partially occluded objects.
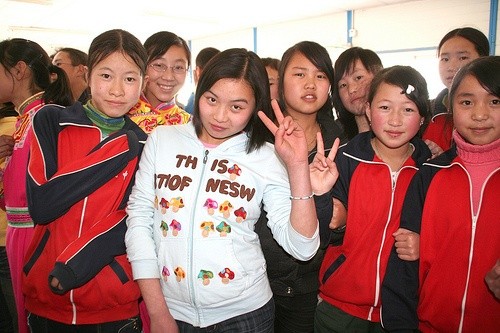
375, 138, 410, 172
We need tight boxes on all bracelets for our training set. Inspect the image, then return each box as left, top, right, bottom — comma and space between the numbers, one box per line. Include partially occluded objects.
290, 192, 315, 200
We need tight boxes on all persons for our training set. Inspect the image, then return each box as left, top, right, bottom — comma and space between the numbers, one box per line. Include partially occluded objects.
0, 27, 500, 333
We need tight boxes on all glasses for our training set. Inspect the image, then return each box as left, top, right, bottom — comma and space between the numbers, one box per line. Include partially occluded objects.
148, 63, 188, 74
52, 60, 78, 67
